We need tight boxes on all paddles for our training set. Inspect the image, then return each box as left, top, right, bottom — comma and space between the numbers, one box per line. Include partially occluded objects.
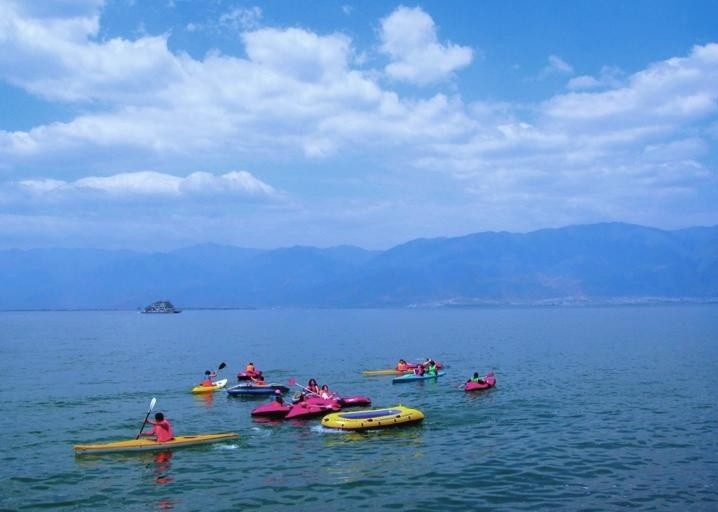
458, 372, 494, 388
137, 397, 156, 440
288, 377, 320, 397
200, 362, 226, 386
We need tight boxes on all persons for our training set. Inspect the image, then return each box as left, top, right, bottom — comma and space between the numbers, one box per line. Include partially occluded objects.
414, 359, 437, 376
246, 362, 255, 373
300, 378, 332, 401
397, 359, 407, 371
203, 370, 216, 386
467, 372, 487, 384
275, 389, 292, 406
251, 375, 265, 386
138, 413, 172, 442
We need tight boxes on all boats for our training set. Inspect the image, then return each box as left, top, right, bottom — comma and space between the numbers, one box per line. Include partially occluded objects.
362, 359, 443, 376
191, 379, 228, 393
323, 430, 424, 444
252, 397, 371, 418
465, 371, 497, 391
285, 397, 342, 418
237, 369, 263, 381
226, 383, 290, 397
321, 405, 425, 429
73, 432, 241, 453
393, 372, 446, 383
